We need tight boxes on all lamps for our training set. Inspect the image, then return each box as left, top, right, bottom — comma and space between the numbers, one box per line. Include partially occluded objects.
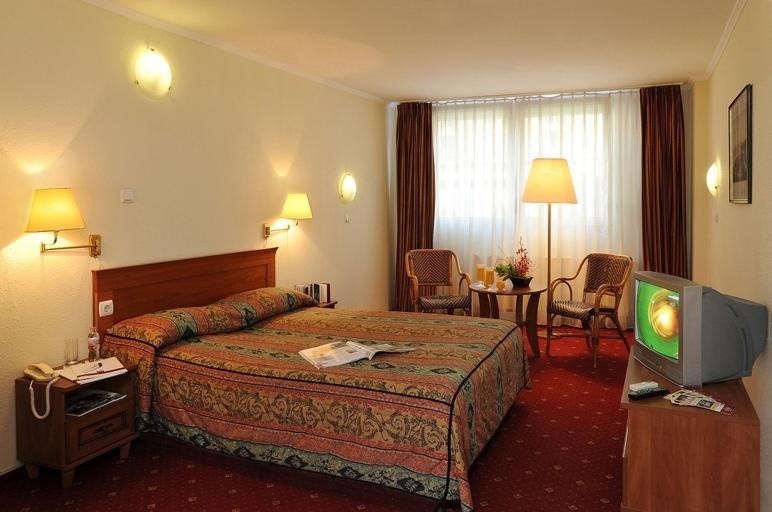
263, 192, 313, 239
521, 158, 578, 340
25, 187, 101, 256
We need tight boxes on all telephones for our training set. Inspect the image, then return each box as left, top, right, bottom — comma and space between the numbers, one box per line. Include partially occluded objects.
23, 362, 59, 382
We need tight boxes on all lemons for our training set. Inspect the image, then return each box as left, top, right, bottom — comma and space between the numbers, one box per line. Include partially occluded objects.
495, 281, 505, 290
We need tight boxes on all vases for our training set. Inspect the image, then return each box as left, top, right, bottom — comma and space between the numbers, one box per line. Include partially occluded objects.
509, 276, 533, 288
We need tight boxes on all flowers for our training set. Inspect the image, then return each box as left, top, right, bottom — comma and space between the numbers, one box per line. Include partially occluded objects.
494, 236, 536, 281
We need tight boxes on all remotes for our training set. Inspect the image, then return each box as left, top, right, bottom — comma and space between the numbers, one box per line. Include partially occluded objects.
628, 387, 670, 401
629, 381, 658, 392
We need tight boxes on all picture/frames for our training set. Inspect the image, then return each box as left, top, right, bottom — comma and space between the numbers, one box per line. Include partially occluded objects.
729, 83, 752, 203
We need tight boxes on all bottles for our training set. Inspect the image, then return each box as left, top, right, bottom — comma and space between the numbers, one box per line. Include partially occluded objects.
88, 329, 99, 362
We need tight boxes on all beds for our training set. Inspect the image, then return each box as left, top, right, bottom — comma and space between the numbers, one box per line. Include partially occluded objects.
92, 246, 527, 506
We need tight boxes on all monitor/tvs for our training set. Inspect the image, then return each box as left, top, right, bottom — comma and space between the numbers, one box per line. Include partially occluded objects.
633, 270, 769, 388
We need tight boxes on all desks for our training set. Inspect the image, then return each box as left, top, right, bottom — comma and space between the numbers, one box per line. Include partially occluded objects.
468, 280, 548, 358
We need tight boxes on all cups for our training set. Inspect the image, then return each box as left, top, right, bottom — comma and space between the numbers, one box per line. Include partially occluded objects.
64, 337, 78, 364
477, 264, 485, 284
497, 275, 506, 291
484, 267, 494, 287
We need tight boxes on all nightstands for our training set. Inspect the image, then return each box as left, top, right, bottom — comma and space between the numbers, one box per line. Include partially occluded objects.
16, 359, 139, 489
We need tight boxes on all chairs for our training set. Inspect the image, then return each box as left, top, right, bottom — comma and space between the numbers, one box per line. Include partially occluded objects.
546, 253, 633, 368
405, 249, 471, 316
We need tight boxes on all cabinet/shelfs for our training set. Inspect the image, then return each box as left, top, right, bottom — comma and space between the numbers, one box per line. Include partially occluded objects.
620, 345, 760, 512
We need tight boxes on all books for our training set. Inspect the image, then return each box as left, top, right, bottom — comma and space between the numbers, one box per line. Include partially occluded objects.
64, 389, 126, 418
72, 355, 131, 380
56, 370, 104, 386
297, 339, 417, 370
293, 282, 331, 304
